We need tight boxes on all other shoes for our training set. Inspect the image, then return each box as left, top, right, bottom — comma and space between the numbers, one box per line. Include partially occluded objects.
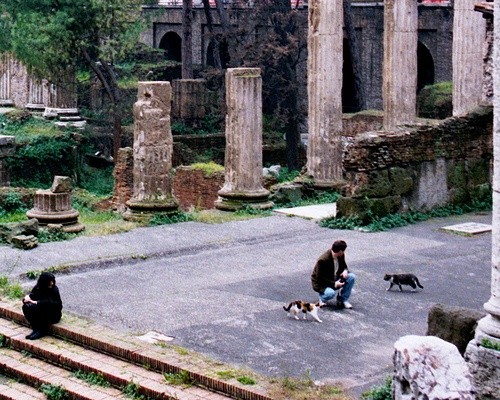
26, 330, 45, 340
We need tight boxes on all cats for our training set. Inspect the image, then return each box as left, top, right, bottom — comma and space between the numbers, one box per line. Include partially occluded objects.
283, 298, 327, 323
383, 274, 424, 292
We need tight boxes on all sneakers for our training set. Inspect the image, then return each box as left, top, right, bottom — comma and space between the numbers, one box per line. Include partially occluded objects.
319, 297, 327, 306
343, 301, 352, 308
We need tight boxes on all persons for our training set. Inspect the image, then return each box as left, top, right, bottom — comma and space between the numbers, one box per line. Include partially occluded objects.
21, 272, 63, 340
311, 240, 356, 309
